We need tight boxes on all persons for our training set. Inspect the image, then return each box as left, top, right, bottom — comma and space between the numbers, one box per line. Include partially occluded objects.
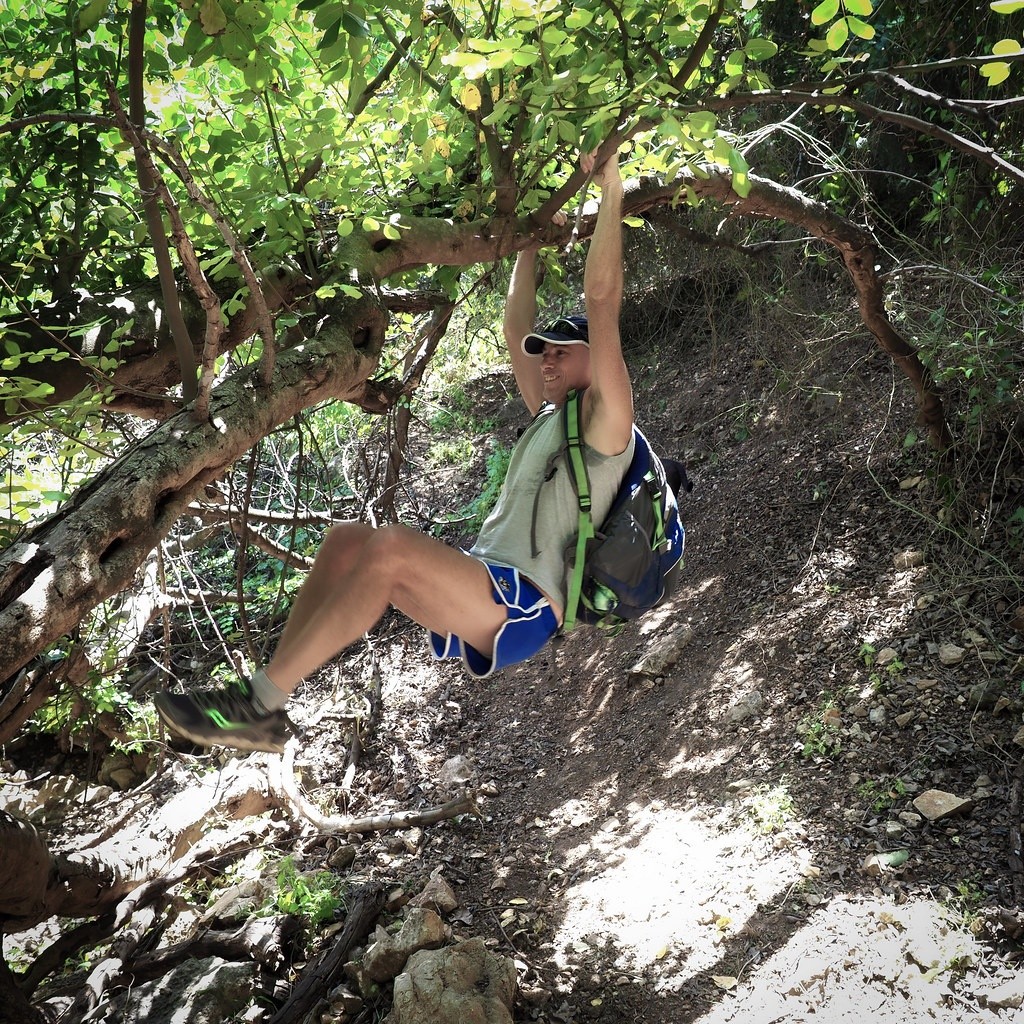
155, 140, 687, 758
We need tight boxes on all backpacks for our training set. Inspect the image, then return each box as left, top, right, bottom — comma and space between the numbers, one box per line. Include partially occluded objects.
516, 385, 694, 638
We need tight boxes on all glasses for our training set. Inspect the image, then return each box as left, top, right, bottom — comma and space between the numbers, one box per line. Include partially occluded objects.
541, 319, 583, 339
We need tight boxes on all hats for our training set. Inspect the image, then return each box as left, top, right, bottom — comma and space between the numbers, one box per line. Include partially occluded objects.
520, 315, 590, 358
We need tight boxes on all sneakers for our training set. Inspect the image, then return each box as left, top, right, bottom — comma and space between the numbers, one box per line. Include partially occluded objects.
153, 675, 287, 753
